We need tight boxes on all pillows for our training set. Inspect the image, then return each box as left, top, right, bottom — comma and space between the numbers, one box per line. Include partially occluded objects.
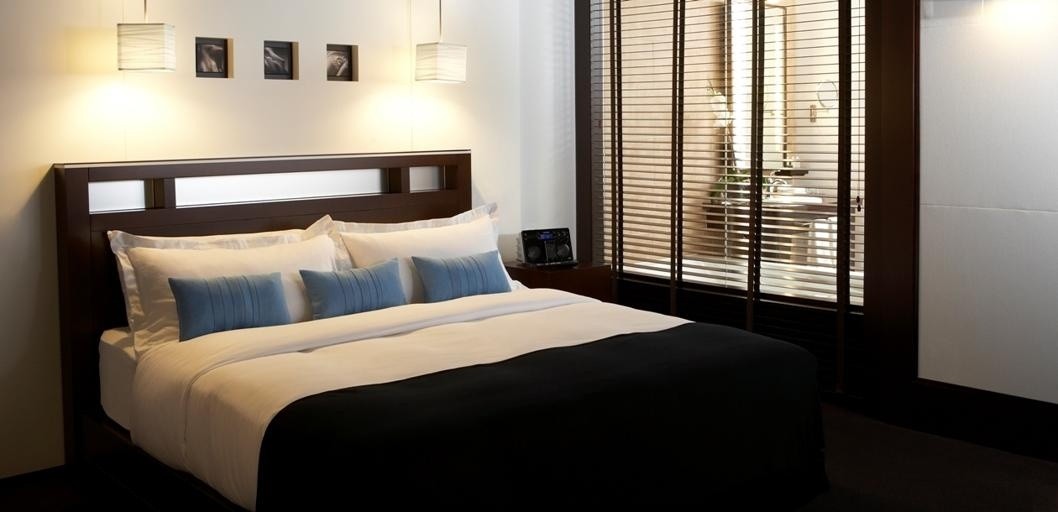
412, 250, 512, 302
331, 201, 516, 305
298, 256, 408, 321
106, 215, 357, 356
169, 273, 294, 341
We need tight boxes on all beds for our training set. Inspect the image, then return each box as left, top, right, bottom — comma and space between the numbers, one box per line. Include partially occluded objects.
52, 149, 832, 512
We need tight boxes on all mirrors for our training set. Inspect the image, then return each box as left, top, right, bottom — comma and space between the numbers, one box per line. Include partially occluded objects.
727, 2, 788, 172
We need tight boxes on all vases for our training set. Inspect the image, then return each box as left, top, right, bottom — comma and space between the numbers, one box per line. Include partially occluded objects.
730, 184, 753, 201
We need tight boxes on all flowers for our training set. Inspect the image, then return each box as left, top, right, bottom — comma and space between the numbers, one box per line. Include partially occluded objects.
704, 80, 770, 200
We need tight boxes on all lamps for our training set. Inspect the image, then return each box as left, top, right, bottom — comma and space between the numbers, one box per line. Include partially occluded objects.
415, 1, 469, 82
117, 1, 176, 73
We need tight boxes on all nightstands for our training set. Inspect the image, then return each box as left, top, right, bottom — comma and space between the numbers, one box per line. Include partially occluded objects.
503, 263, 611, 302
